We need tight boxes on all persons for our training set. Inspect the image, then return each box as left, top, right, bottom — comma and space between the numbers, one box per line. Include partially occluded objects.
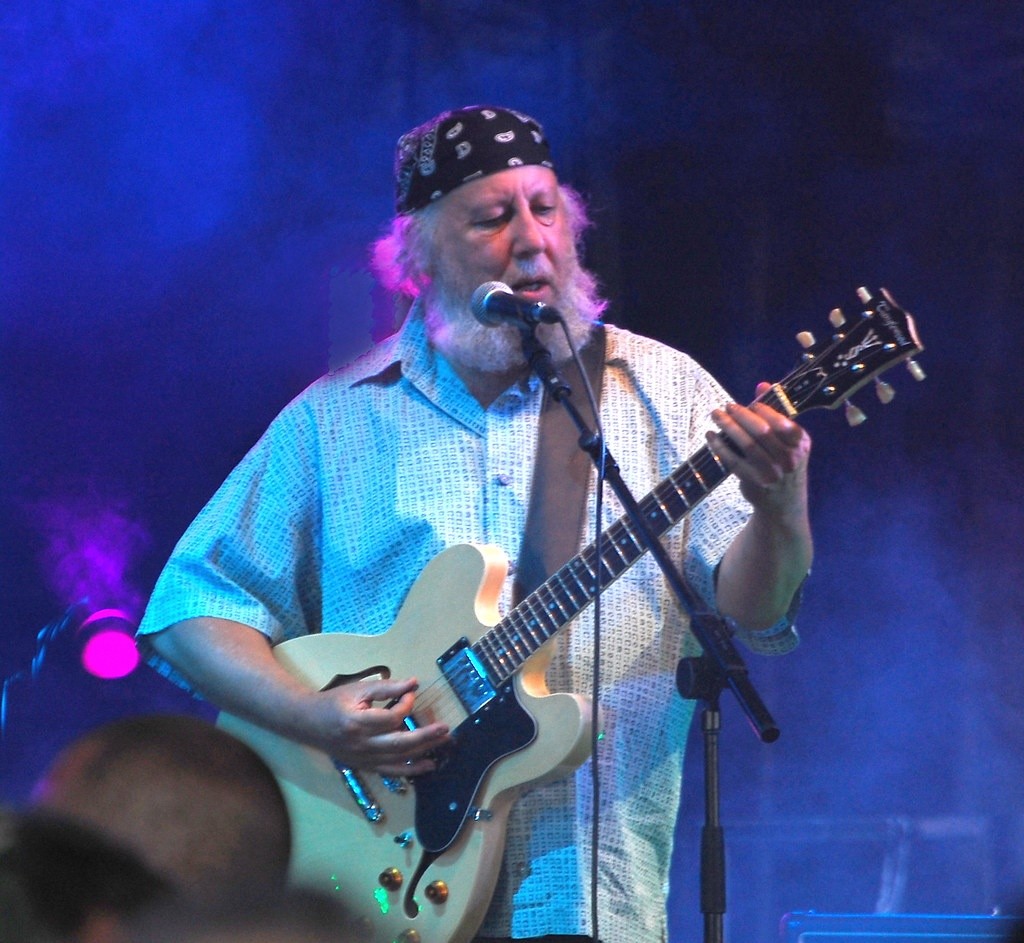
0, 712, 378, 942
133, 96, 826, 939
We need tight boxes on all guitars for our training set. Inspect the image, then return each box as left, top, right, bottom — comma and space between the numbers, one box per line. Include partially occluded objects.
213, 285, 928, 943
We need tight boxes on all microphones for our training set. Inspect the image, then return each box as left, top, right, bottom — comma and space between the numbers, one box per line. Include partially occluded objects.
471, 281, 561, 328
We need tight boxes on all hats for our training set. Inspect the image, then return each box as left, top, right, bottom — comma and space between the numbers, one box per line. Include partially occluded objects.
395, 104, 554, 215
